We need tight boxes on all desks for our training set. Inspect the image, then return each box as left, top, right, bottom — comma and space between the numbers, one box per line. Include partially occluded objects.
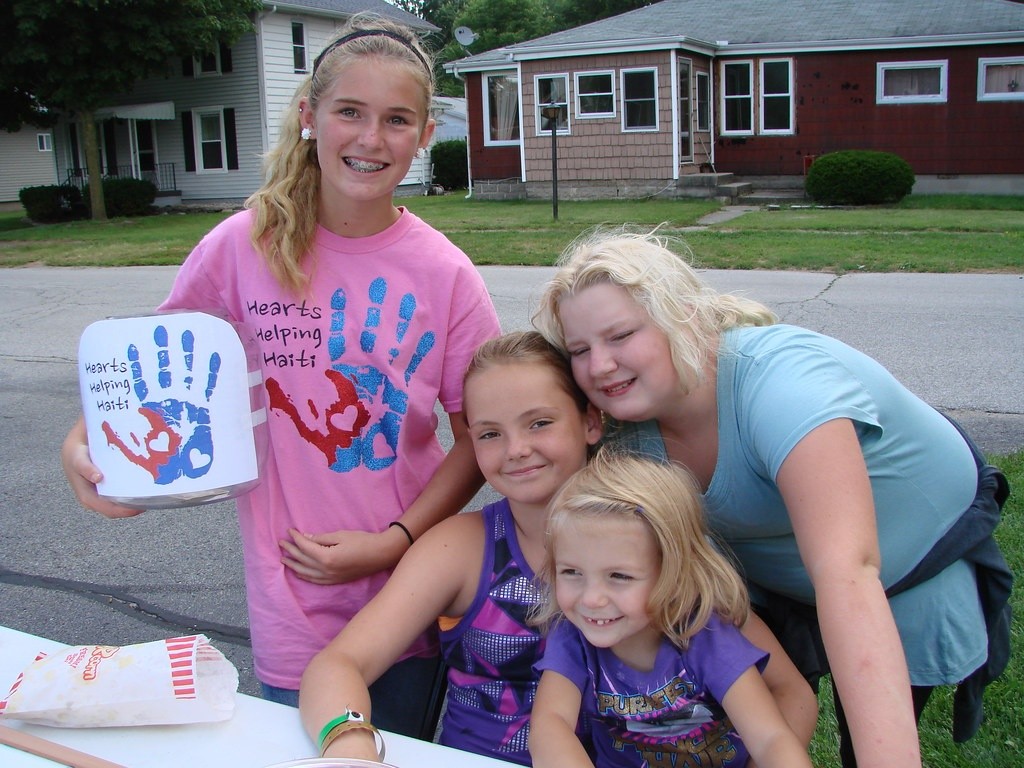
0, 623, 533, 768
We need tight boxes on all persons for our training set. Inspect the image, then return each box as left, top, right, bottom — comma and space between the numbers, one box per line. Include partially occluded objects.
529, 233, 990, 767
525, 452, 814, 768
60, 17, 504, 740
296, 330, 821, 768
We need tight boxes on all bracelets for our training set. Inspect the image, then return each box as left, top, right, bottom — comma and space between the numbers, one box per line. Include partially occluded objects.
389, 522, 414, 545
318, 721, 386, 763
316, 710, 368, 749
